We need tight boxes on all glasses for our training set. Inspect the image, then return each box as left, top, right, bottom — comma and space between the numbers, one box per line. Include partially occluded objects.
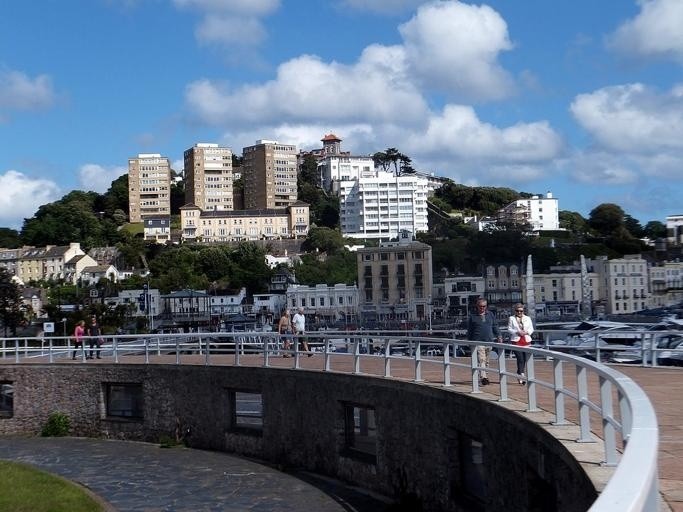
516, 309, 524, 312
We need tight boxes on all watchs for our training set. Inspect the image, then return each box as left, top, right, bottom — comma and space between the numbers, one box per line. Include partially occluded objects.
497, 337, 503, 340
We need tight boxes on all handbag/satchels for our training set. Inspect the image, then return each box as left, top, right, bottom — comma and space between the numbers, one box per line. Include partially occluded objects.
97, 338, 105, 345
281, 328, 287, 340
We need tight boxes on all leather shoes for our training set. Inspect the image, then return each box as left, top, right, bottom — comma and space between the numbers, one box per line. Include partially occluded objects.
482, 377, 490, 385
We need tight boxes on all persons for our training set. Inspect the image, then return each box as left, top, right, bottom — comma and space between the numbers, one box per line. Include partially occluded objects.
467, 298, 503, 386
87, 316, 102, 359
292, 306, 314, 358
507, 302, 534, 387
71, 320, 90, 361
278, 309, 293, 359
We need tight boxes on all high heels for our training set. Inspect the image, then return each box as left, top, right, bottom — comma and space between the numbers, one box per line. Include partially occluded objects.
518, 377, 526, 384
